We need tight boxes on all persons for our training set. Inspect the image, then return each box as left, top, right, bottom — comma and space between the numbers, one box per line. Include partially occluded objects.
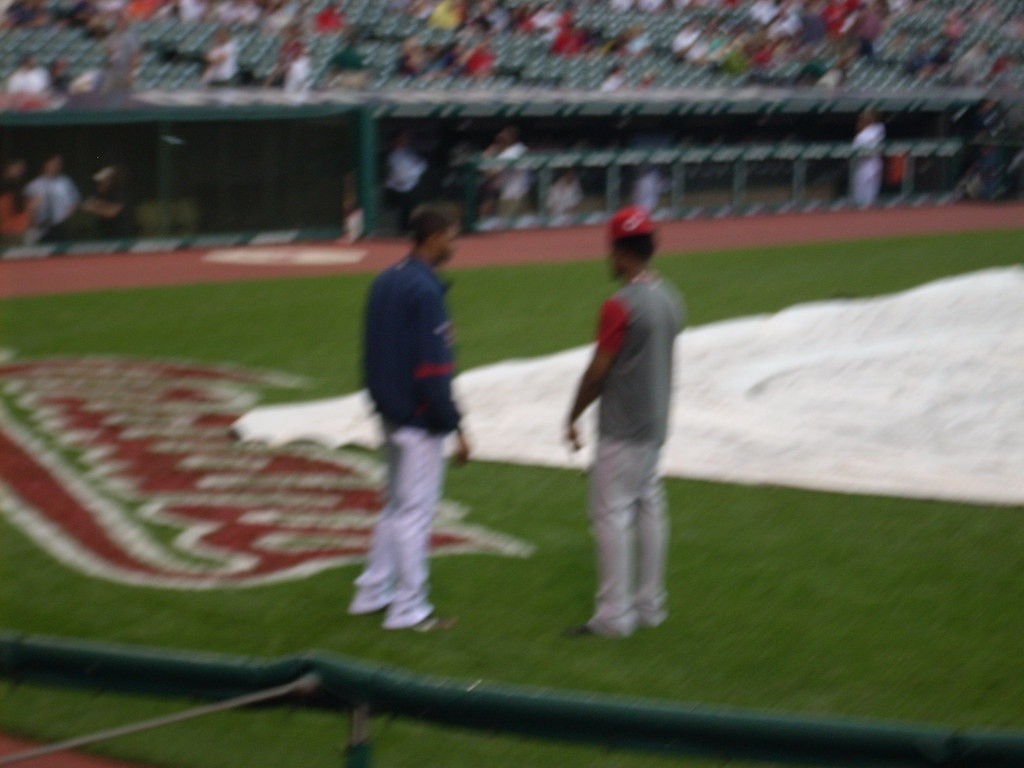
349, 207, 472, 631
635, 169, 670, 211
561, 209, 687, 638
548, 171, 587, 226
387, 130, 530, 231
0, 0, 1024, 94
0, 160, 126, 247
851, 111, 884, 209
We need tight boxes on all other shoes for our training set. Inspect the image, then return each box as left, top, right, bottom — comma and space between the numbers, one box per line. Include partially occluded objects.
347, 592, 450, 632
562, 606, 666, 640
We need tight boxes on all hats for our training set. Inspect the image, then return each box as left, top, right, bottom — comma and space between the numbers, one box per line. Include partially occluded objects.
608, 207, 655, 242
